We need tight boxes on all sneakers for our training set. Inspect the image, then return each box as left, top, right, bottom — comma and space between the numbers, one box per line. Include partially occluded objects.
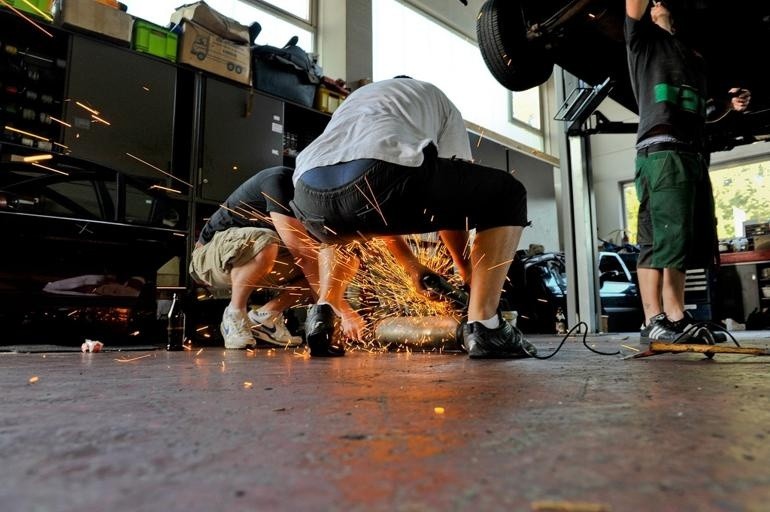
673, 311, 727, 343
219, 305, 257, 349
463, 309, 538, 359
247, 305, 303, 347
304, 303, 345, 357
640, 311, 702, 345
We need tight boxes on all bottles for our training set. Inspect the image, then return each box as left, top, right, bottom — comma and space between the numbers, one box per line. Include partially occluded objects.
167, 292, 186, 351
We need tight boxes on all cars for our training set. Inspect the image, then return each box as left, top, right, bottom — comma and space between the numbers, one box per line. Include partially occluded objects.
41, 275, 156, 342
476, 0, 769, 152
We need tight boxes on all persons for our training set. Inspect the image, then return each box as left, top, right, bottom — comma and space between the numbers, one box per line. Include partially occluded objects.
290, 75, 537, 360
623, 0, 751, 347
188, 168, 367, 350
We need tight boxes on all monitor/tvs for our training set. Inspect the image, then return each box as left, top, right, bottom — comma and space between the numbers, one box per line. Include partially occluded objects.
742, 220, 769, 251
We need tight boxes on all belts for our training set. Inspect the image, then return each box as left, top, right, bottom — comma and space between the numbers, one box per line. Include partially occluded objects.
637, 143, 699, 156
